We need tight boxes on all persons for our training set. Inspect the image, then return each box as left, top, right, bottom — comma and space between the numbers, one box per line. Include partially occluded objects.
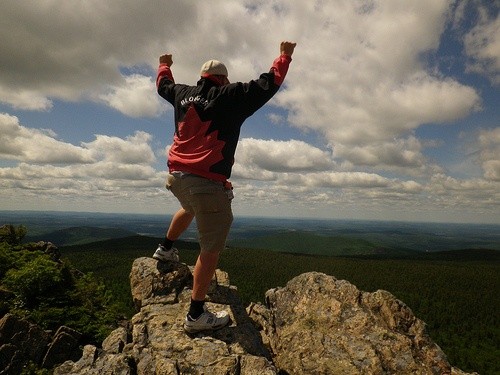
150, 40, 297, 333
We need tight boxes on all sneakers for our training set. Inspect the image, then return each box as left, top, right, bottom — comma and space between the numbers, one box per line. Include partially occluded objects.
153, 243, 180, 263
183, 310, 231, 335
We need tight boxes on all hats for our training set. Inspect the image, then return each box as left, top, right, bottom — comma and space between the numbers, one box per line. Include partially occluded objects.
200, 60, 231, 84
